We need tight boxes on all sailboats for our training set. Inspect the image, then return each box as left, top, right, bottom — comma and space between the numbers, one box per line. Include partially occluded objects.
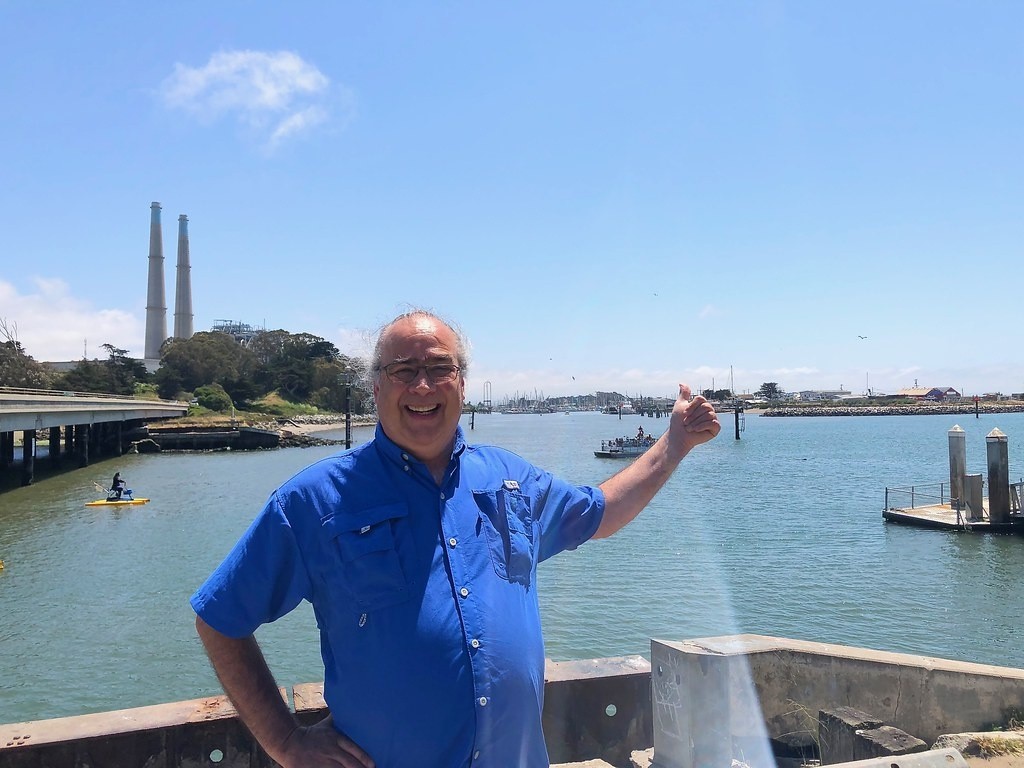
494, 383, 684, 416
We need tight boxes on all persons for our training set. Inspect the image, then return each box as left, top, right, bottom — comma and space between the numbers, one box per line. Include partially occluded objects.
111, 472, 126, 500
609, 429, 654, 446
188, 303, 722, 768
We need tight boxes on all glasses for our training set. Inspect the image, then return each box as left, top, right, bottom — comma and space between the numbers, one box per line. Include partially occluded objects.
380, 362, 462, 384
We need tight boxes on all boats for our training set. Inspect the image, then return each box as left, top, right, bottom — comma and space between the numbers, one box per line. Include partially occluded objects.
593, 424, 659, 458
